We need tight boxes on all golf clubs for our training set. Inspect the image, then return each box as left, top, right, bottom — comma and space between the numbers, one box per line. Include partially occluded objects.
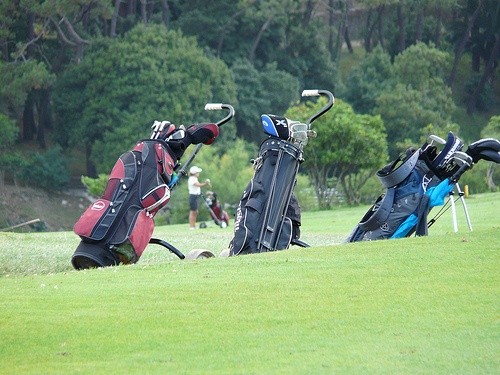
261, 112, 317, 144
149, 120, 185, 144
420, 132, 499, 180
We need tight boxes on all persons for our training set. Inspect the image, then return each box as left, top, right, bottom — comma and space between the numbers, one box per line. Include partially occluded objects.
187, 166, 210, 230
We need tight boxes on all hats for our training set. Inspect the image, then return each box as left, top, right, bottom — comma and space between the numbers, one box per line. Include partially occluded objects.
190, 166, 202, 174
